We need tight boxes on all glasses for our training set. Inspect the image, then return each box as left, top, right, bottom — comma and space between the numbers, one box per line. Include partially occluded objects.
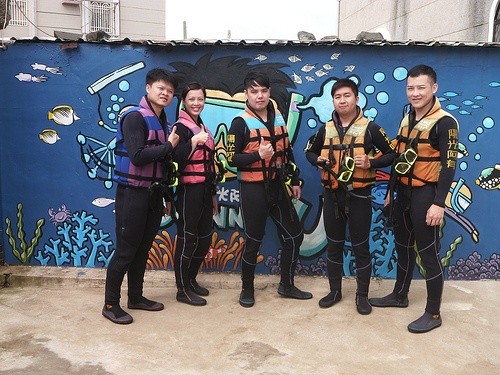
218, 161, 226, 184
169, 162, 179, 187
336, 157, 355, 183
284, 160, 296, 186
394, 148, 417, 175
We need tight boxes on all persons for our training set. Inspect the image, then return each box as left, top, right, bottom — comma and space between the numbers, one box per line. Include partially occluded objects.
305, 78, 398, 315
102, 69, 180, 325
170, 82, 218, 306
368, 63, 459, 333
227, 71, 314, 307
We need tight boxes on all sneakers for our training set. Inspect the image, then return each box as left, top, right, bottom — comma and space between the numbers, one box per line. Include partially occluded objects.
368, 291, 409, 308
356, 291, 372, 315
102, 304, 133, 325
239, 284, 255, 307
128, 295, 164, 311
319, 289, 342, 308
277, 283, 313, 299
176, 288, 207, 305
407, 311, 442, 333
185, 274, 209, 296
